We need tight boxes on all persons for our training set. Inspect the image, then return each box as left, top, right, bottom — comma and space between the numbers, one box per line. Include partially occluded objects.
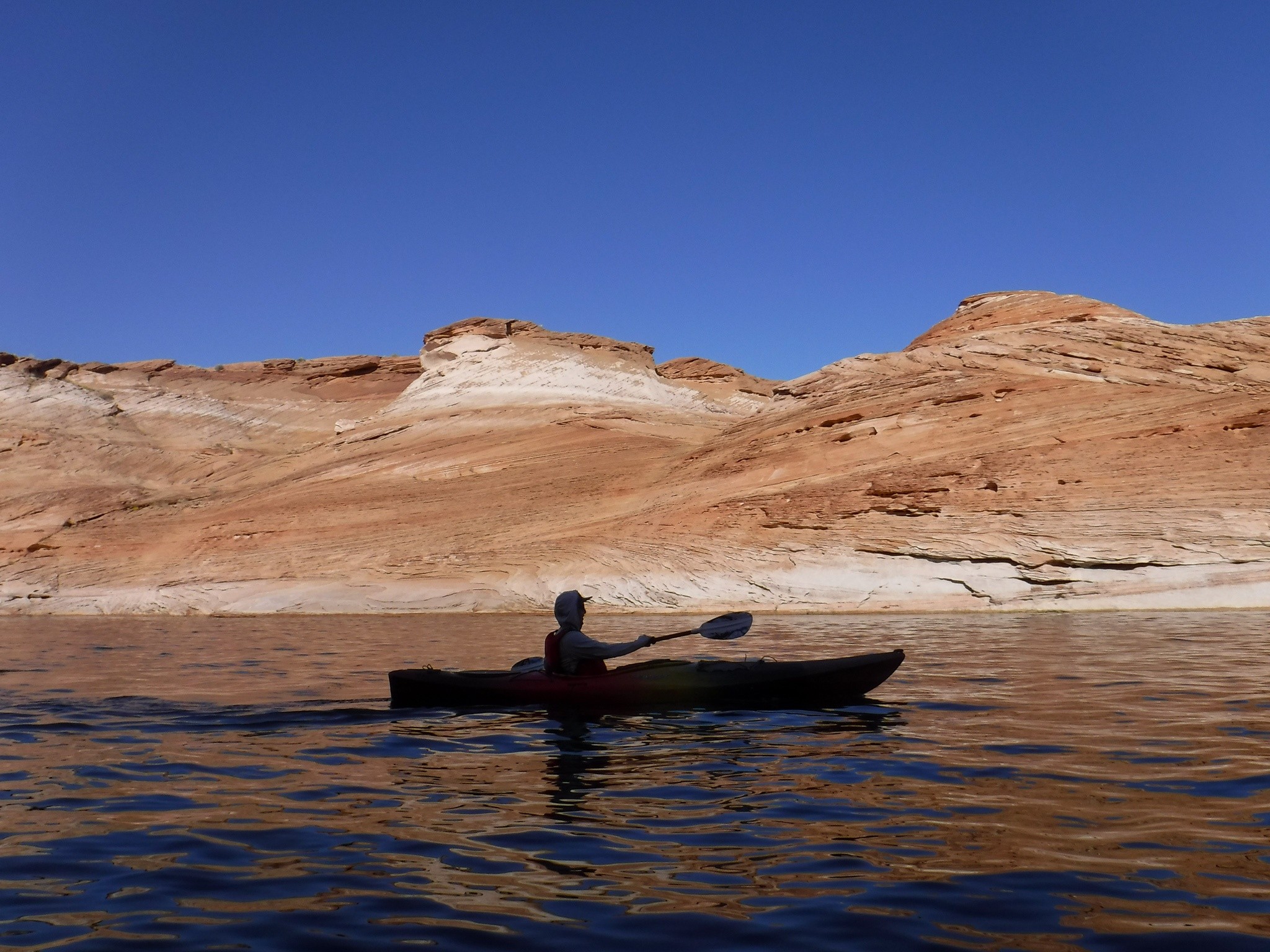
544, 590, 653, 676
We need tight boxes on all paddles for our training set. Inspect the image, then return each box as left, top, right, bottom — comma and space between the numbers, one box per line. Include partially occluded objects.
511, 611, 753, 674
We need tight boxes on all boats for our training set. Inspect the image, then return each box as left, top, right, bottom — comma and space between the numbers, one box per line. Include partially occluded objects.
388, 648, 907, 713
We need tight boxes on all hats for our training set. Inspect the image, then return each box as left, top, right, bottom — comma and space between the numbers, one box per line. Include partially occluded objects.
578, 592, 593, 603
